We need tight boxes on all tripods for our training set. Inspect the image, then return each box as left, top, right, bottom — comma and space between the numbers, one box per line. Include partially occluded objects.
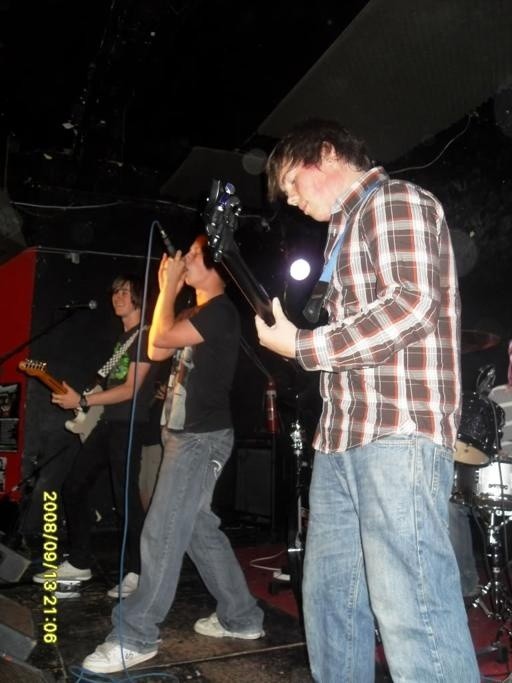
466, 526, 512, 623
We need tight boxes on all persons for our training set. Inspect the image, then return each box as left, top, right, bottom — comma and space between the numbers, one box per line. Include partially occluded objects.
50, 268, 173, 603
80, 233, 265, 677
447, 341, 512, 609
254, 119, 481, 683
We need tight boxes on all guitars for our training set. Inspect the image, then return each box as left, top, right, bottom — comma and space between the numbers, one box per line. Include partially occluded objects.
18, 358, 104, 446
203, 183, 324, 445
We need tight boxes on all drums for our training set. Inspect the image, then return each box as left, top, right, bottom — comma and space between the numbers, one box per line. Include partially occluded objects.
457, 391, 504, 469
450, 460, 477, 506
471, 455, 512, 515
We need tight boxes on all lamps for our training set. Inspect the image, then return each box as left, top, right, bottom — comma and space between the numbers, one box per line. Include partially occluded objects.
286, 251, 312, 281
42, 115, 79, 161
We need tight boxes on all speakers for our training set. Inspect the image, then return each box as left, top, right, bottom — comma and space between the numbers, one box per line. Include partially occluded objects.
0, 530, 33, 584
0, 594, 39, 663
0, 650, 51, 683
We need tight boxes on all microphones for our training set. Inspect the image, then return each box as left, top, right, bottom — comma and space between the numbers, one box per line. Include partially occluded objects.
65, 300, 98, 310
478, 367, 496, 398
158, 224, 176, 259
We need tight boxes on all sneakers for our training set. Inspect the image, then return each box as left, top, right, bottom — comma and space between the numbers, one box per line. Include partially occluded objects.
82, 641, 158, 674
33, 560, 92, 583
107, 572, 138, 597
192, 612, 261, 640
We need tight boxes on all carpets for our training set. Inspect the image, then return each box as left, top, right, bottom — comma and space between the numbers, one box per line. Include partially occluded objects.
232, 544, 512, 683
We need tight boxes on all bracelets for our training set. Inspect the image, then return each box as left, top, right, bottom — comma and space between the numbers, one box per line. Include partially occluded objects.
80, 395, 89, 413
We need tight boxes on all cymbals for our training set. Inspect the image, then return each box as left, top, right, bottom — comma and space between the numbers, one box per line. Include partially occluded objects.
462, 328, 503, 356
